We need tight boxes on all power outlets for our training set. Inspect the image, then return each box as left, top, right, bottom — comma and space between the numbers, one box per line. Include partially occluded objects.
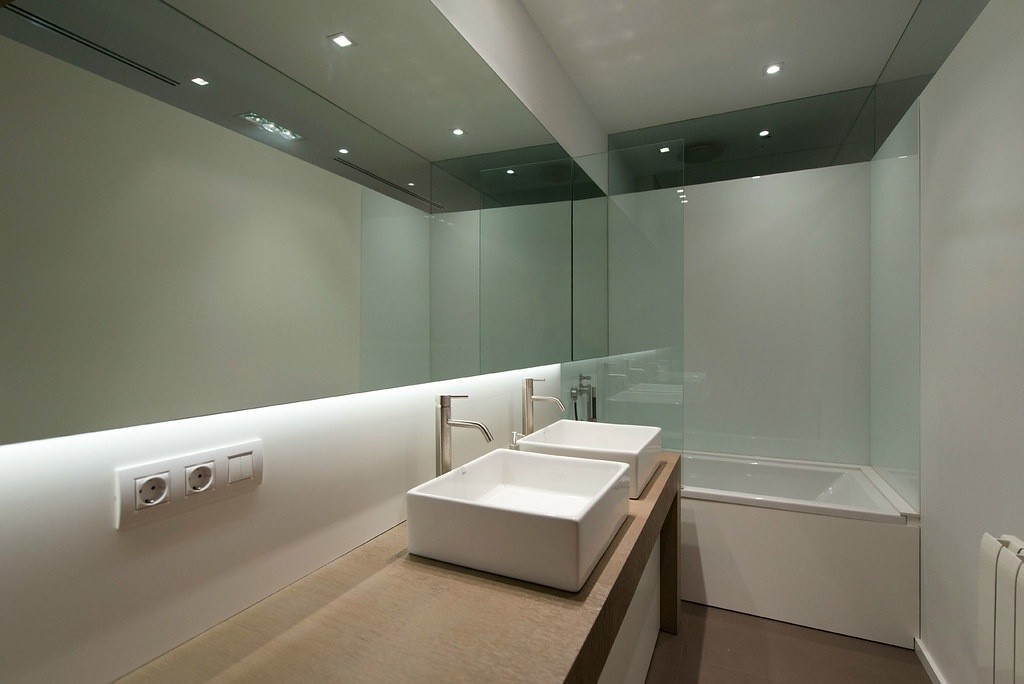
115, 450, 218, 530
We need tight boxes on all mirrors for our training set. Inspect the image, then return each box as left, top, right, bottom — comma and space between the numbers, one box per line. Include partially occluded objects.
0, 37, 608, 443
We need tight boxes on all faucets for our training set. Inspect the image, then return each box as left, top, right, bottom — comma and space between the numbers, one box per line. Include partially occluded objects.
434, 394, 494, 478
603, 362, 630, 398
521, 376, 567, 438
622, 358, 646, 391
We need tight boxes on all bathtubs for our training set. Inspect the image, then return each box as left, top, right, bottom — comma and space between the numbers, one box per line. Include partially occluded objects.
662, 448, 921, 652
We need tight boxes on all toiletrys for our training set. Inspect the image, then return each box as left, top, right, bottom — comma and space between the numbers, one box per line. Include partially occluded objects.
508, 430, 526, 450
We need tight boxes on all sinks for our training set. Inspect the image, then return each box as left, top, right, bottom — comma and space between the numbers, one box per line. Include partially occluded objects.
515, 418, 663, 499
405, 447, 631, 593
627, 383, 683, 393
605, 391, 683, 422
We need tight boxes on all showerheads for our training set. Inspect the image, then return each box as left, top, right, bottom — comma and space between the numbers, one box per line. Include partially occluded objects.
591, 386, 597, 419
